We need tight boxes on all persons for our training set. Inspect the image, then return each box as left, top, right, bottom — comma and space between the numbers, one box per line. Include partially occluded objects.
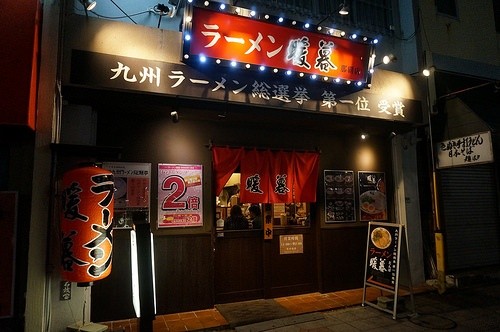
215, 188, 305, 230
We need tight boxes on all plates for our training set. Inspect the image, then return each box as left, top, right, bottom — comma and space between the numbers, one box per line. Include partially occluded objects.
114, 178, 126, 199
371, 227, 392, 249
360, 191, 386, 215
377, 177, 385, 193
326, 187, 353, 195
325, 175, 353, 183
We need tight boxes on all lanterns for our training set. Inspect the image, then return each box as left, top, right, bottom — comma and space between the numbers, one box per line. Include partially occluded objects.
58, 166, 114, 287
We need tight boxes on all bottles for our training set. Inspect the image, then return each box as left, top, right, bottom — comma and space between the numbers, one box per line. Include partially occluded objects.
280, 212, 287, 226
